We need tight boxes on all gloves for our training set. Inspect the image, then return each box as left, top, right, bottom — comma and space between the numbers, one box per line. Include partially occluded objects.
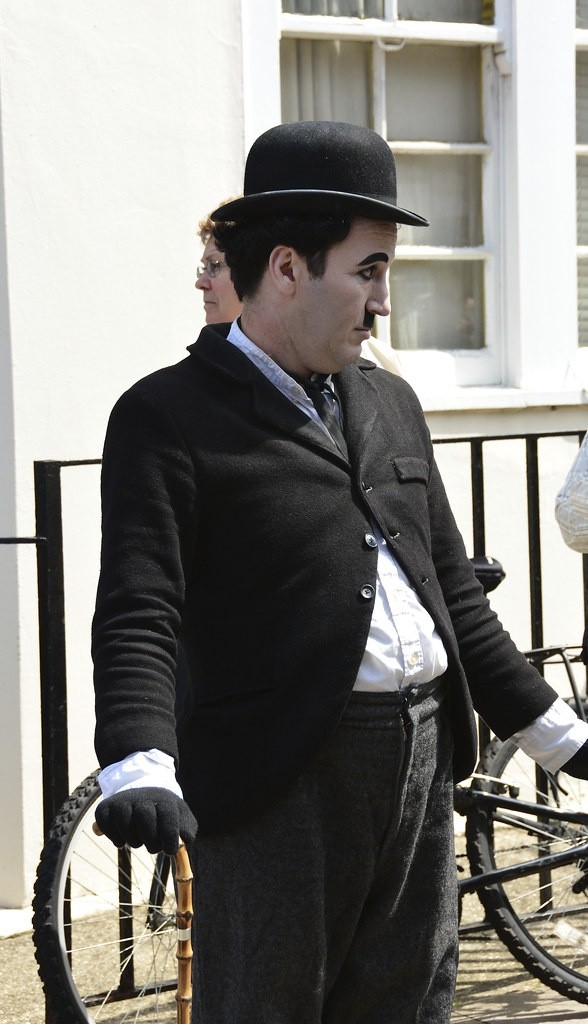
560, 738, 588, 782
94, 788, 200, 856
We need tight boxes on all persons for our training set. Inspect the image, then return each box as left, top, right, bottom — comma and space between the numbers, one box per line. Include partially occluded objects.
193, 203, 408, 396
86, 125, 587, 1024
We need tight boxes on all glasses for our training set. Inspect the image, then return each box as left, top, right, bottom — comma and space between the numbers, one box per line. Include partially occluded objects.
196, 261, 228, 277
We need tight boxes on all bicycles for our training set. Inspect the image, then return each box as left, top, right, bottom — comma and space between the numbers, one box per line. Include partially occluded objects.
30, 556, 587, 1023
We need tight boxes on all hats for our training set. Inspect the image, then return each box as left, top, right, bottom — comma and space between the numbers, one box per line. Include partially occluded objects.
209, 120, 430, 226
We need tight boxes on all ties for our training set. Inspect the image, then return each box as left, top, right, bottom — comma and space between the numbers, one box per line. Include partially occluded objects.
288, 371, 351, 462
